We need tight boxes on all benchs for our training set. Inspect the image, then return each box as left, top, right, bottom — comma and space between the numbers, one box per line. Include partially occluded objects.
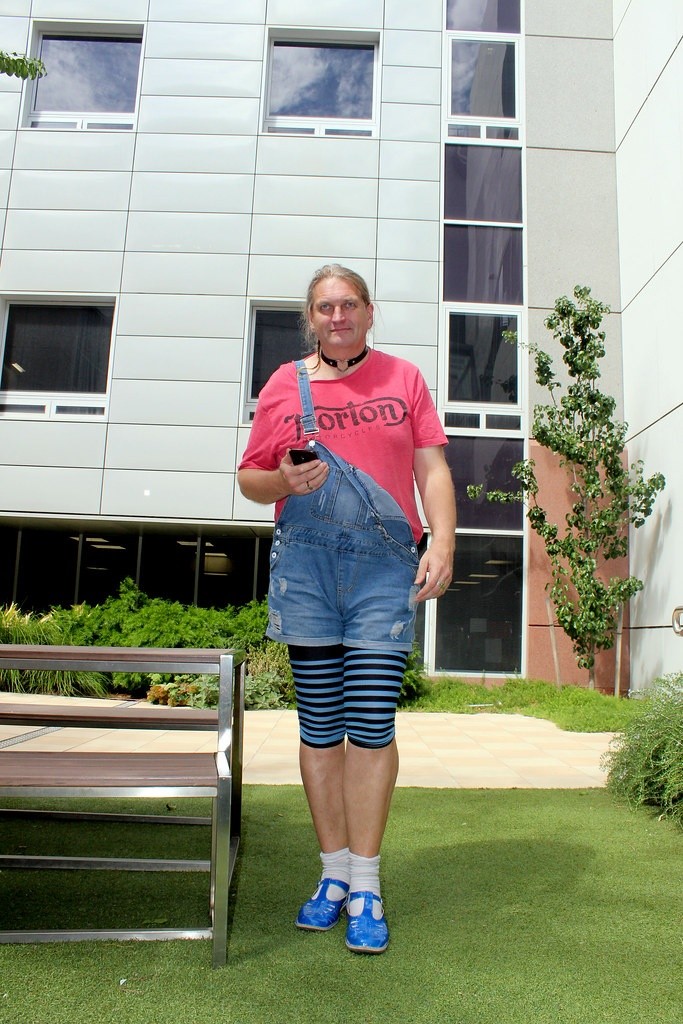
0, 643, 244, 968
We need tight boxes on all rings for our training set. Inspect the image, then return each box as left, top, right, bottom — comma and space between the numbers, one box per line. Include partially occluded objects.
306, 481, 313, 490
437, 581, 446, 591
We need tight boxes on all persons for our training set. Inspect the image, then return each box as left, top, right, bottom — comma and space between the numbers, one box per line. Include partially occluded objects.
237, 264, 458, 954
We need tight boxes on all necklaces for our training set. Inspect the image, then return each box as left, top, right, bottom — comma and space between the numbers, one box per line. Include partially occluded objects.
321, 345, 368, 372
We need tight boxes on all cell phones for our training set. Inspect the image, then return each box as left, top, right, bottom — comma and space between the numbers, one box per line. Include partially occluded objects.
289, 449, 318, 465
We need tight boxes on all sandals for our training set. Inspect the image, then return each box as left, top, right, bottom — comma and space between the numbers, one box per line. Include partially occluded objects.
345, 890, 389, 954
295, 878, 350, 932
